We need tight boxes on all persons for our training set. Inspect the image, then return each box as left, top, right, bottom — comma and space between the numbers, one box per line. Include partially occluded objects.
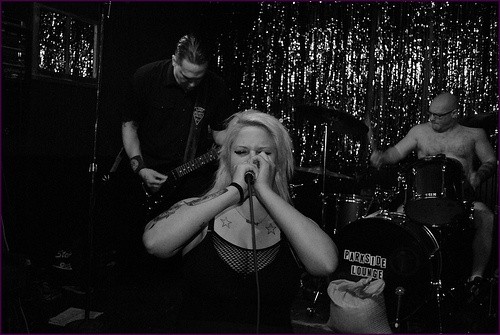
371, 94, 496, 295
120, 33, 234, 334
143, 109, 338, 334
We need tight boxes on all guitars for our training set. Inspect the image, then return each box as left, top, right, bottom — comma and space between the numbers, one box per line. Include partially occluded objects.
87, 139, 221, 230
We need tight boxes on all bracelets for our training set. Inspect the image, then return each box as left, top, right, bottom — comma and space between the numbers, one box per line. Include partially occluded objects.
129, 155, 146, 174
229, 182, 244, 205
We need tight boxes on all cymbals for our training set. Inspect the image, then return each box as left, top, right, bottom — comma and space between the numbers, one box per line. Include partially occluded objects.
295, 101, 371, 134
293, 165, 357, 181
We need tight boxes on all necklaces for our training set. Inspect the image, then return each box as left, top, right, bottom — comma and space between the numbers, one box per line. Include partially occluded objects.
233, 205, 269, 224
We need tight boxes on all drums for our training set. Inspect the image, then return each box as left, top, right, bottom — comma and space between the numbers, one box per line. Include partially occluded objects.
402, 153, 468, 226
329, 208, 441, 312
319, 192, 375, 230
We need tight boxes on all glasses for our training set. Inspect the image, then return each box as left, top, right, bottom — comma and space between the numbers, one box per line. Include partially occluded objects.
425, 109, 457, 120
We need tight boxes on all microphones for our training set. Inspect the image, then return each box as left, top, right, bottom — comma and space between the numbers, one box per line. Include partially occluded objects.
244, 170, 255, 184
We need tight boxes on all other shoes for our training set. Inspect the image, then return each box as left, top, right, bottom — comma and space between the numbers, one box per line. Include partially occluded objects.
464, 277, 490, 312
